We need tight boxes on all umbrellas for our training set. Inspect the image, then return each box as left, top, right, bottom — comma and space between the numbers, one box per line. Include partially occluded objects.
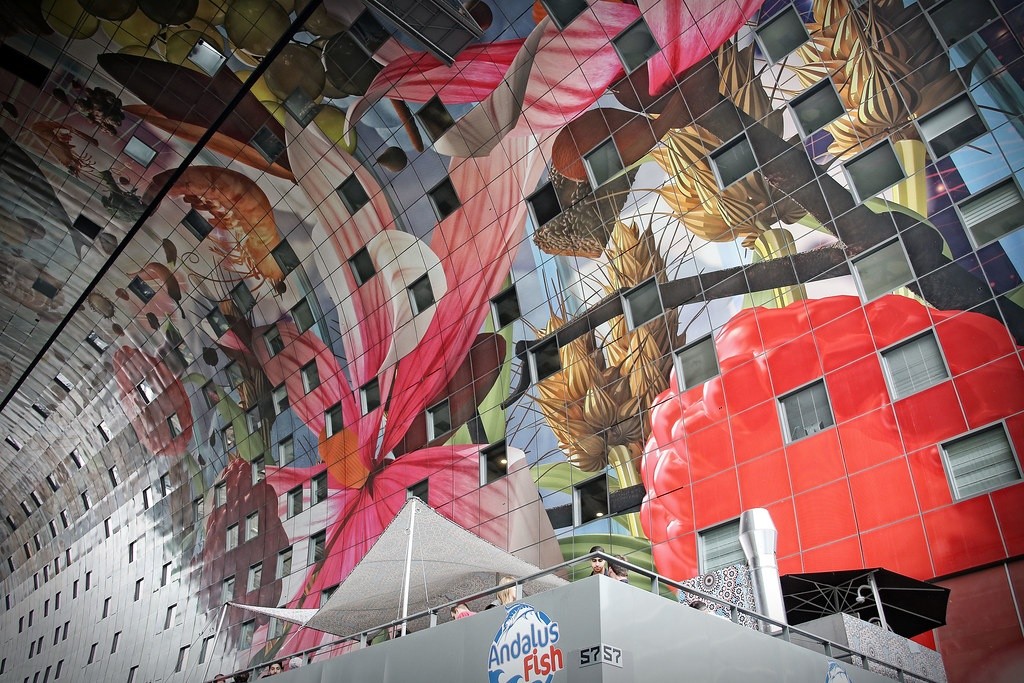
780, 567, 951, 639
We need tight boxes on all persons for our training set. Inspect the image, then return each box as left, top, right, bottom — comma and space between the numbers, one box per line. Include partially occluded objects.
289, 657, 302, 669
689, 601, 707, 612
485, 577, 517, 610
390, 620, 411, 639
261, 661, 283, 679
590, 546, 629, 584
451, 603, 477, 620
213, 669, 249, 683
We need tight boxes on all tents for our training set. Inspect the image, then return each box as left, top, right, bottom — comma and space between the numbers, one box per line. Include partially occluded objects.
164, 496, 571, 683
